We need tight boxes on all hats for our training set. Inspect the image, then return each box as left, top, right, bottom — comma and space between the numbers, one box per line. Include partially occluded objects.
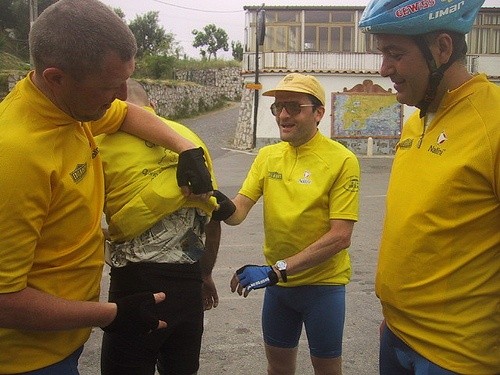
262, 73, 327, 105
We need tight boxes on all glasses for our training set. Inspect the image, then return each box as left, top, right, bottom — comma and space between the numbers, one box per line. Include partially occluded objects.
270, 101, 316, 116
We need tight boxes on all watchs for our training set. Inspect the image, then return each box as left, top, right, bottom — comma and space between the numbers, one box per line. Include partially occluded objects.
275, 260, 288, 282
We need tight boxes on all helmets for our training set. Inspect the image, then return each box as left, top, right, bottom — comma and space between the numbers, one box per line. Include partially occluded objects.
358, 0, 486, 36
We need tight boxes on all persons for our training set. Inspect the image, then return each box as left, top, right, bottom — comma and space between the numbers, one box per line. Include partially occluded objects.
358, 0, 500, 375
212, 73, 361, 375
92, 77, 221, 374
0, 0, 215, 375
